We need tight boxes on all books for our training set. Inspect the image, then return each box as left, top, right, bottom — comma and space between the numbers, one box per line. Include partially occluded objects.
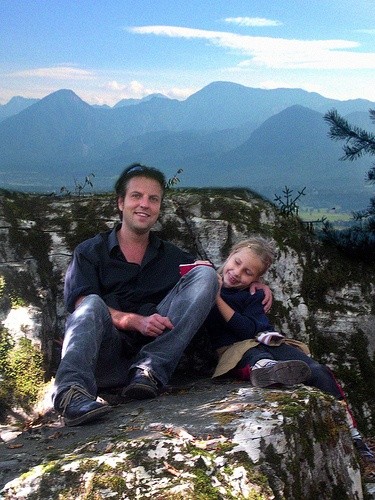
178, 261, 212, 276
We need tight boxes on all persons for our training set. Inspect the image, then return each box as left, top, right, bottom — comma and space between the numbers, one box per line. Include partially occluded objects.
193, 236, 373, 459
51, 163, 219, 427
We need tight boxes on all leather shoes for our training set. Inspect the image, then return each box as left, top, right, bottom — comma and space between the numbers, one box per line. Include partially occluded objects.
54, 388, 113, 426
122, 369, 158, 399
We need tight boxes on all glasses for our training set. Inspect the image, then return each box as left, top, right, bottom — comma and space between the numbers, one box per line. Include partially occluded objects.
124, 166, 146, 182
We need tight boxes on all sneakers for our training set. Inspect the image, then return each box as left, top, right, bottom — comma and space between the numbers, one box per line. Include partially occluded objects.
353, 438, 374, 462
249, 359, 311, 388
